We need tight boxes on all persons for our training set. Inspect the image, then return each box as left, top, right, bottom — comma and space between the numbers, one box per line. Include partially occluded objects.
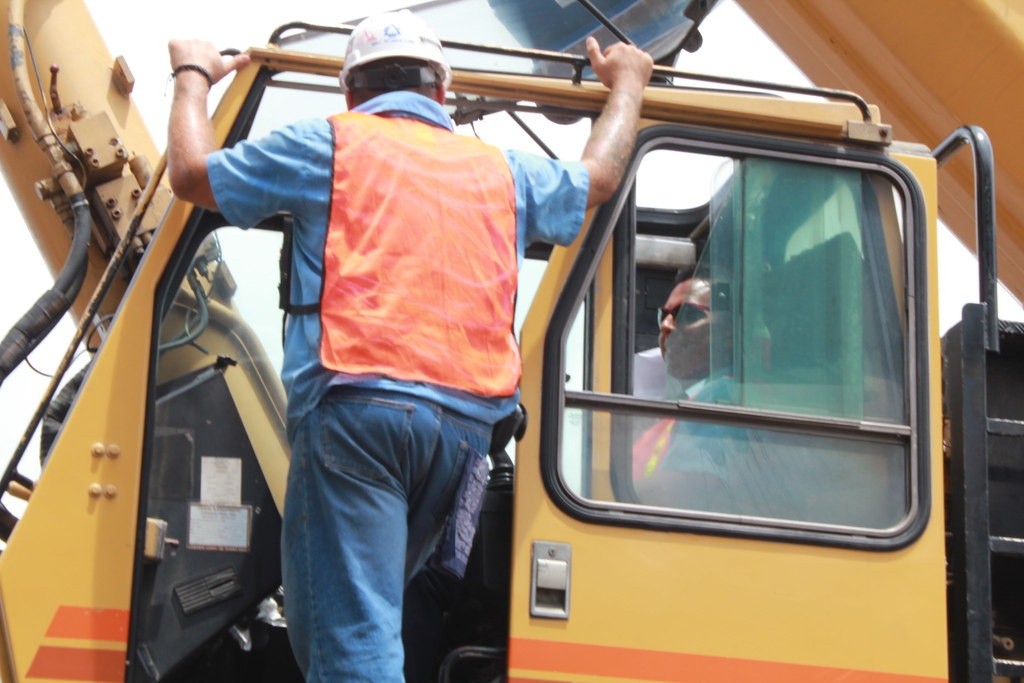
168, 9, 654, 683
631, 262, 790, 515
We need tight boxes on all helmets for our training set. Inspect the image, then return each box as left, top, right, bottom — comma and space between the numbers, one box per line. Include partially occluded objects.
341, 8, 453, 93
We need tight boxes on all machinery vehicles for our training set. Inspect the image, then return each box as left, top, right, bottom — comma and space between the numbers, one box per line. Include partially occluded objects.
0, 0, 1024, 683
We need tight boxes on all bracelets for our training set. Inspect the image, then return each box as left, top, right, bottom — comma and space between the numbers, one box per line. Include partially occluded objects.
174, 64, 213, 86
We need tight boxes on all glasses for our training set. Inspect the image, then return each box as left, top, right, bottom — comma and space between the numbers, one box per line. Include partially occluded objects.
657, 303, 709, 328
